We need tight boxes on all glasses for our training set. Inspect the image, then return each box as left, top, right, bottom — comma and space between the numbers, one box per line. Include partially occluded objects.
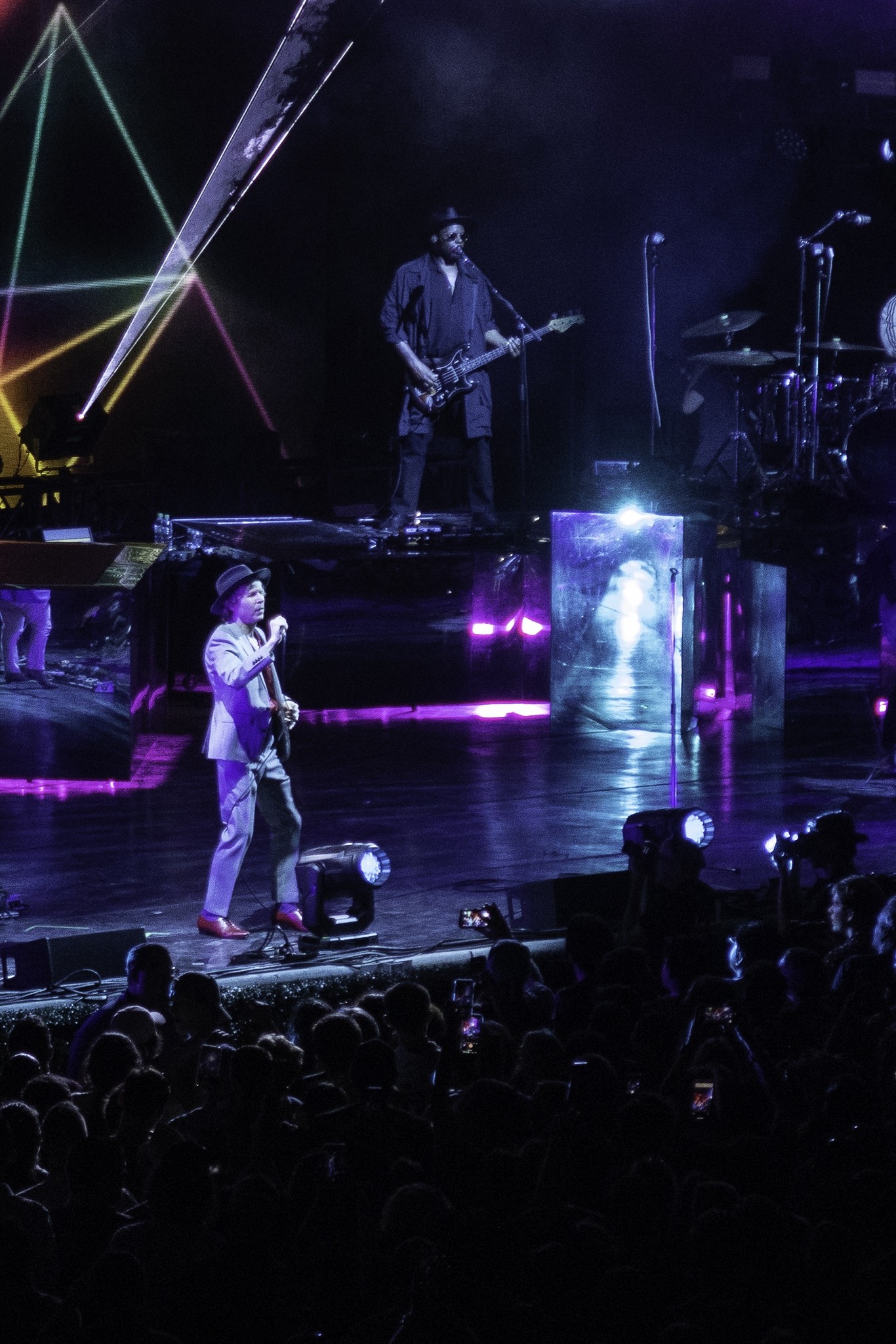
436, 232, 468, 243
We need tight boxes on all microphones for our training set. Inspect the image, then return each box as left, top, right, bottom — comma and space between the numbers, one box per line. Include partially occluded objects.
854, 216, 872, 225
453, 245, 474, 267
280, 626, 286, 637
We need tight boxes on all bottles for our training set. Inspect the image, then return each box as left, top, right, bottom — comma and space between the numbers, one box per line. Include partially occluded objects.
154, 513, 172, 547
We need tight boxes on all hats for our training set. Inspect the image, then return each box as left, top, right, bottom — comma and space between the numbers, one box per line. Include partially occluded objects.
432, 203, 472, 234
210, 564, 271, 615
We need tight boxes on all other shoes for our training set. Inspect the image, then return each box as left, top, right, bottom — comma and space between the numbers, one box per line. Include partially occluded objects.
4, 672, 28, 684
469, 510, 510, 532
380, 512, 417, 533
25, 668, 59, 689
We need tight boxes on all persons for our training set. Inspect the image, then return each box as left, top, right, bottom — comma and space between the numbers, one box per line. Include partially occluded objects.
379, 216, 524, 531
196, 564, 310, 938
0, 589, 58, 688
0, 811, 896, 1344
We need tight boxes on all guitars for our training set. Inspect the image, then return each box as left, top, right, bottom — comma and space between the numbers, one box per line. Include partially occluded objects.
408, 308, 586, 418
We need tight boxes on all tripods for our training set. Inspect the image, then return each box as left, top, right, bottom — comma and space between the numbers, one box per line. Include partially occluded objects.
699, 365, 769, 499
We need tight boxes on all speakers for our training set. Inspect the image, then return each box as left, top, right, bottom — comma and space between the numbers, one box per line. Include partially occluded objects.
506, 870, 630, 932
0, 928, 147, 991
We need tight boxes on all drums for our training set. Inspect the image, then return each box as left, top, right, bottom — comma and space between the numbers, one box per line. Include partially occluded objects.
840, 403, 896, 506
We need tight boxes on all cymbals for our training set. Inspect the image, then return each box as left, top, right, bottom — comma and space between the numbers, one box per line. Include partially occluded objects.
685, 351, 808, 367
681, 311, 763, 337
790, 342, 886, 350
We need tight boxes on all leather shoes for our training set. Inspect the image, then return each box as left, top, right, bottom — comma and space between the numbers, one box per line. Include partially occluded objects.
197, 914, 251, 939
270, 907, 309, 934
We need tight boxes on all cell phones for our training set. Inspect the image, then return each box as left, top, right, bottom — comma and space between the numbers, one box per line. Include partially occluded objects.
454, 978, 475, 1018
460, 1014, 483, 1054
691, 1080, 714, 1120
459, 909, 491, 929
